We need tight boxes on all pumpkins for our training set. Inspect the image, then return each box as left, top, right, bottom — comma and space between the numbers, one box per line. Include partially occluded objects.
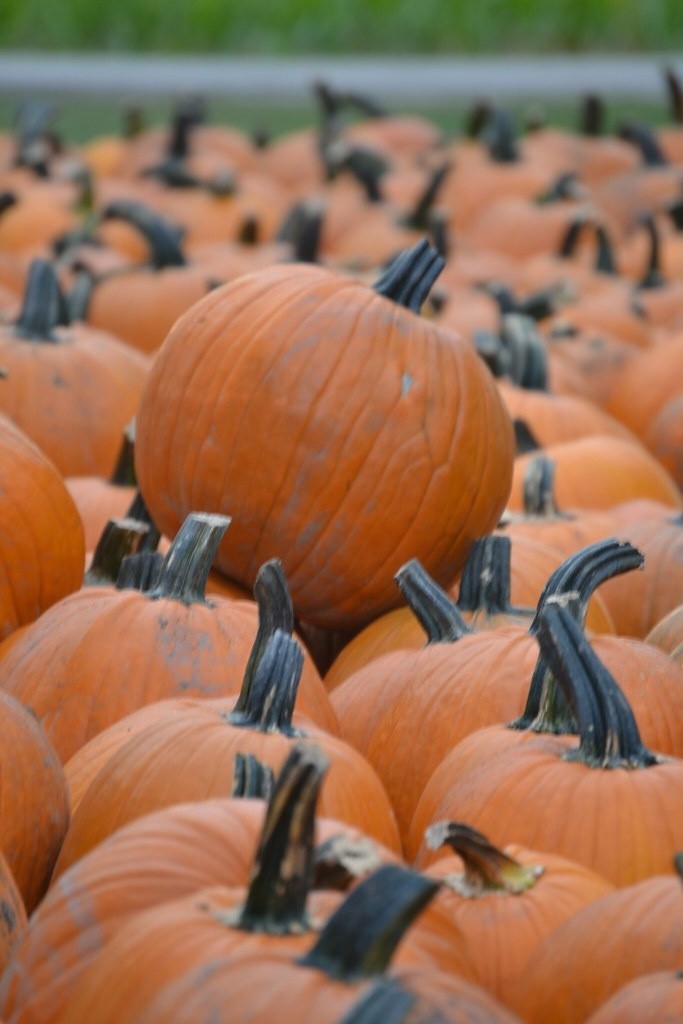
0, 62, 683, 1024
